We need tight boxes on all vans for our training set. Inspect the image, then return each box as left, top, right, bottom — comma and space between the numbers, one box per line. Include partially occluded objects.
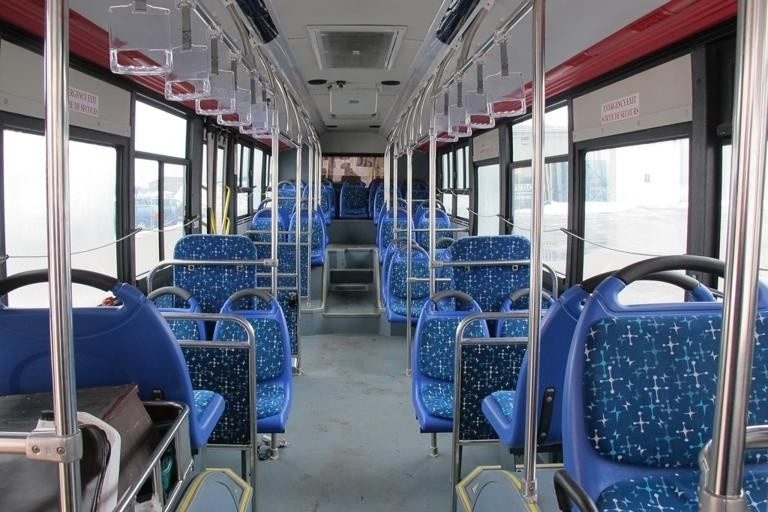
132, 195, 178, 230
